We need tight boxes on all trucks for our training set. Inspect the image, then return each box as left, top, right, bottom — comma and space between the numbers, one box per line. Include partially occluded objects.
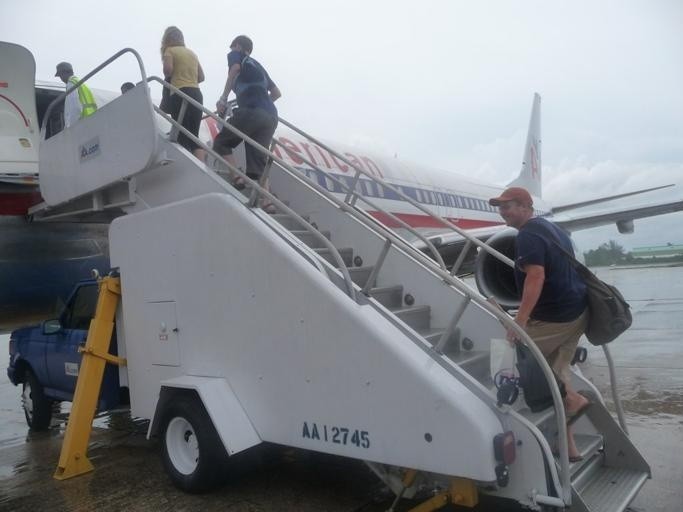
6, 269, 264, 494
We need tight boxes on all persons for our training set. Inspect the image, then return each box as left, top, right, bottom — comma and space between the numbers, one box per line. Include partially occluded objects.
121, 82, 135, 94
488, 187, 598, 464
55, 62, 98, 131
212, 34, 282, 214
160, 25, 207, 165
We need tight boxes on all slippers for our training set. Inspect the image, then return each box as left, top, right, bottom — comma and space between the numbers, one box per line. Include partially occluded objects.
552, 400, 596, 439
550, 445, 583, 462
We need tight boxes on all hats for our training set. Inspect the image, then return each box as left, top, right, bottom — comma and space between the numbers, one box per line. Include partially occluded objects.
55, 64, 73, 78
489, 187, 533, 206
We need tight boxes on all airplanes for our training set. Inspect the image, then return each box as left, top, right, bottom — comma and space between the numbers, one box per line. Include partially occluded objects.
0, 40, 682, 335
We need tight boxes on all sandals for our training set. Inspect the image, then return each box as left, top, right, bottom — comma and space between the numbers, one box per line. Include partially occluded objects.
229, 175, 245, 188
259, 203, 276, 213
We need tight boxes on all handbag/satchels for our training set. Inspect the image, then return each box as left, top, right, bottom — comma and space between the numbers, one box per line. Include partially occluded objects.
160, 81, 173, 113
585, 275, 633, 345
